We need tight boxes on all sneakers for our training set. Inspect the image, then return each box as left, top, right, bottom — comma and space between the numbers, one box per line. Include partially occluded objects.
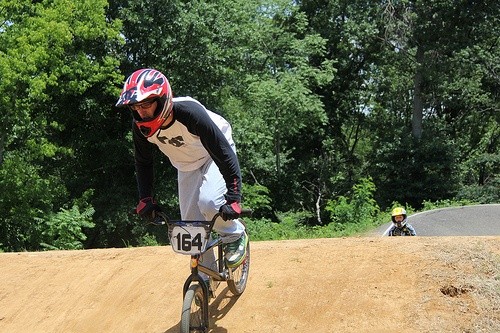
195, 281, 211, 306
224, 230, 248, 268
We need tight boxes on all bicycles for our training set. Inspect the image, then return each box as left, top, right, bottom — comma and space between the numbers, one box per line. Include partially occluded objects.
139, 212, 250, 333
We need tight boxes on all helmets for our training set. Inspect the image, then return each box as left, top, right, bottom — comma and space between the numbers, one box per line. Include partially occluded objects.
115, 69, 173, 138
391, 207, 408, 228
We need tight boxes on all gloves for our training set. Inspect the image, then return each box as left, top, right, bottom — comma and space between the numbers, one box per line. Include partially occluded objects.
137, 196, 155, 223
219, 200, 241, 221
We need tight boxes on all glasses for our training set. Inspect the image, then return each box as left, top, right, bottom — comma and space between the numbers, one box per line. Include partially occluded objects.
130, 98, 157, 111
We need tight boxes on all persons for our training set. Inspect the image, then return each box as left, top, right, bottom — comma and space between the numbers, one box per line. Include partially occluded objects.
388, 207, 417, 237
114, 68, 249, 307
